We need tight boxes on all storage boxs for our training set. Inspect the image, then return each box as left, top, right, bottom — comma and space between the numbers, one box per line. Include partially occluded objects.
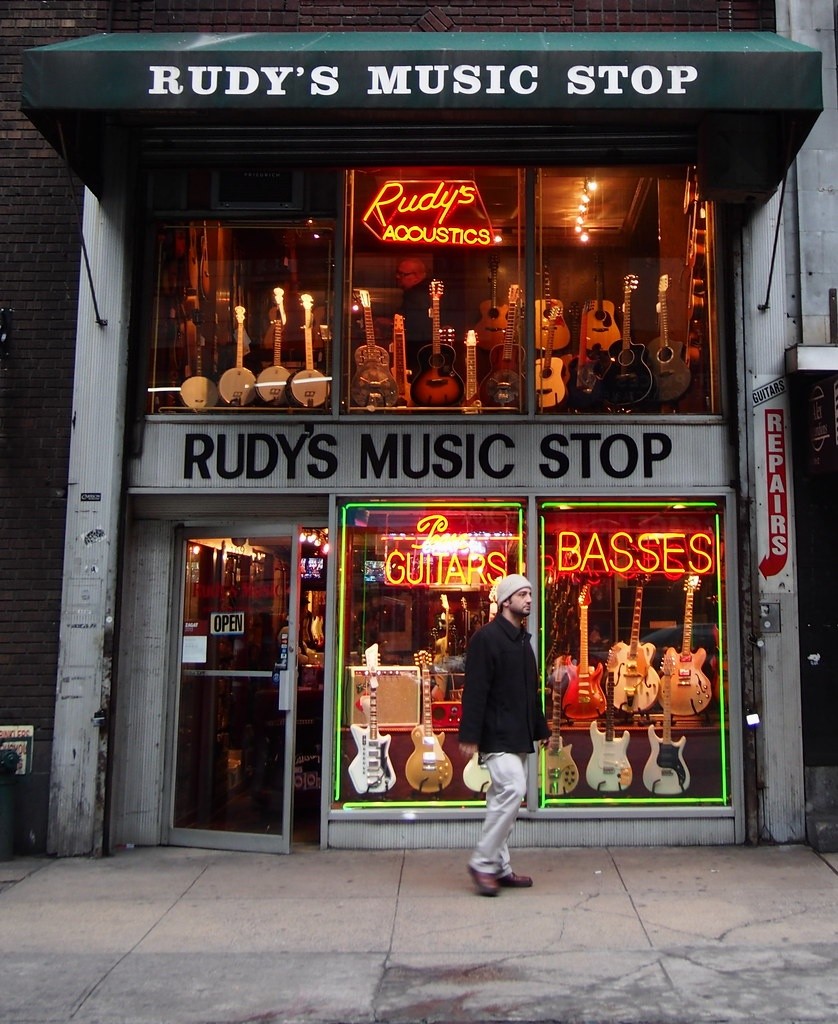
343, 665, 421, 728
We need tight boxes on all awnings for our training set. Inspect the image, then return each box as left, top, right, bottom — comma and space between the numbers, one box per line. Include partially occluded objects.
18, 30, 826, 204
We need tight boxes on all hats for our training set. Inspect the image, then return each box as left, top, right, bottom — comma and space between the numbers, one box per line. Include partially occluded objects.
497, 574, 531, 607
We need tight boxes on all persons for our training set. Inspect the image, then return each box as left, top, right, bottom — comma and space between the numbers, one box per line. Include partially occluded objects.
396, 257, 435, 364
458, 574, 552, 897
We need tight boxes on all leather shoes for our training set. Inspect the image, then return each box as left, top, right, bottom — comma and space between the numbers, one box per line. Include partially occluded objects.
466, 863, 499, 896
496, 871, 533, 887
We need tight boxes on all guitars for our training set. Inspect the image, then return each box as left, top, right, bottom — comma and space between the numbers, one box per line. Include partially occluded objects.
657, 573, 711, 717
462, 750, 493, 793
584, 645, 634, 792
347, 643, 398, 796
563, 583, 608, 723
404, 650, 454, 795
605, 571, 661, 712
641, 650, 691, 796
174, 264, 694, 417
537, 656, 579, 796
431, 591, 487, 652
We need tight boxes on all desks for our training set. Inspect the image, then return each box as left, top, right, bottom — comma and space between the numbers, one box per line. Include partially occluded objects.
546, 724, 731, 807
338, 727, 526, 809
